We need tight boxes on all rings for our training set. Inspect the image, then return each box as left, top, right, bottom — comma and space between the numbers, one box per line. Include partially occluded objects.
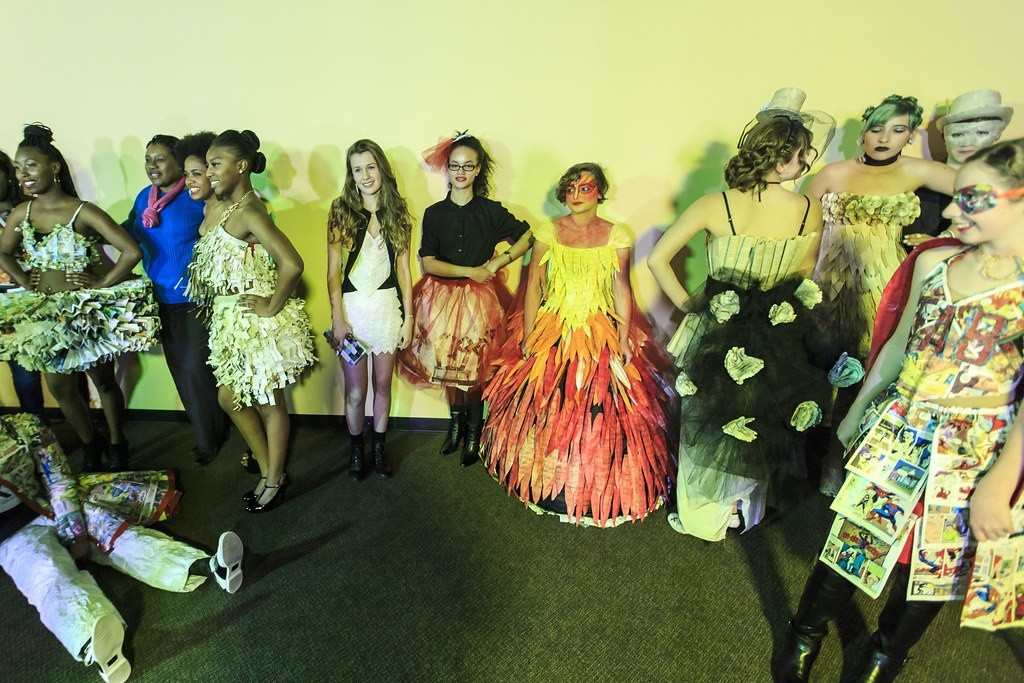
247, 302, 250, 308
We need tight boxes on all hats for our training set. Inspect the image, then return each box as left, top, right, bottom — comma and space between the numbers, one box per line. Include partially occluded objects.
756, 88, 808, 124
936, 89, 1014, 131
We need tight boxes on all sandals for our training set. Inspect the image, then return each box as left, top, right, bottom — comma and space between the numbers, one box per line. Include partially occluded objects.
814, 455, 844, 498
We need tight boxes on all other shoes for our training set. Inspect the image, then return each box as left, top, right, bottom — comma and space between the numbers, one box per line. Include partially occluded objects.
194, 439, 218, 467
110, 440, 130, 477
82, 440, 101, 475
241, 449, 260, 472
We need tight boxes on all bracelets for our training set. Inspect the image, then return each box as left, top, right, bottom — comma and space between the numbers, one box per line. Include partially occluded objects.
937, 230, 955, 238
504, 250, 512, 262
404, 315, 413, 317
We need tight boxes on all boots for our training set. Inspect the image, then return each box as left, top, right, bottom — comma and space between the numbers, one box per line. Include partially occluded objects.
858, 569, 945, 683
372, 428, 390, 479
460, 393, 484, 466
346, 430, 368, 486
776, 574, 856, 683
439, 406, 465, 456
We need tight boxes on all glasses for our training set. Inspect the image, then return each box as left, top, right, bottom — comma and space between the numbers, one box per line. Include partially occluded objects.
447, 161, 479, 172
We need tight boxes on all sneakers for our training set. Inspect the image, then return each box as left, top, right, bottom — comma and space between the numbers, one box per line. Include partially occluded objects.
209, 532, 242, 593
85, 616, 132, 681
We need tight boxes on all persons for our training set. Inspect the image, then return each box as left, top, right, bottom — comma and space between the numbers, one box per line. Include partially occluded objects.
395, 129, 533, 466
806, 94, 958, 497
778, 138, 1024, 683
900, 90, 1024, 255
647, 88, 861, 542
0, 122, 161, 473
482, 164, 679, 529
0, 152, 90, 427
96, 135, 216, 466
0, 412, 243, 683
173, 129, 317, 511
327, 139, 414, 481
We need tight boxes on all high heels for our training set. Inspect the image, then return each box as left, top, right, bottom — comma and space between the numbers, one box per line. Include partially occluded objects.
243, 476, 269, 502
246, 476, 292, 511
